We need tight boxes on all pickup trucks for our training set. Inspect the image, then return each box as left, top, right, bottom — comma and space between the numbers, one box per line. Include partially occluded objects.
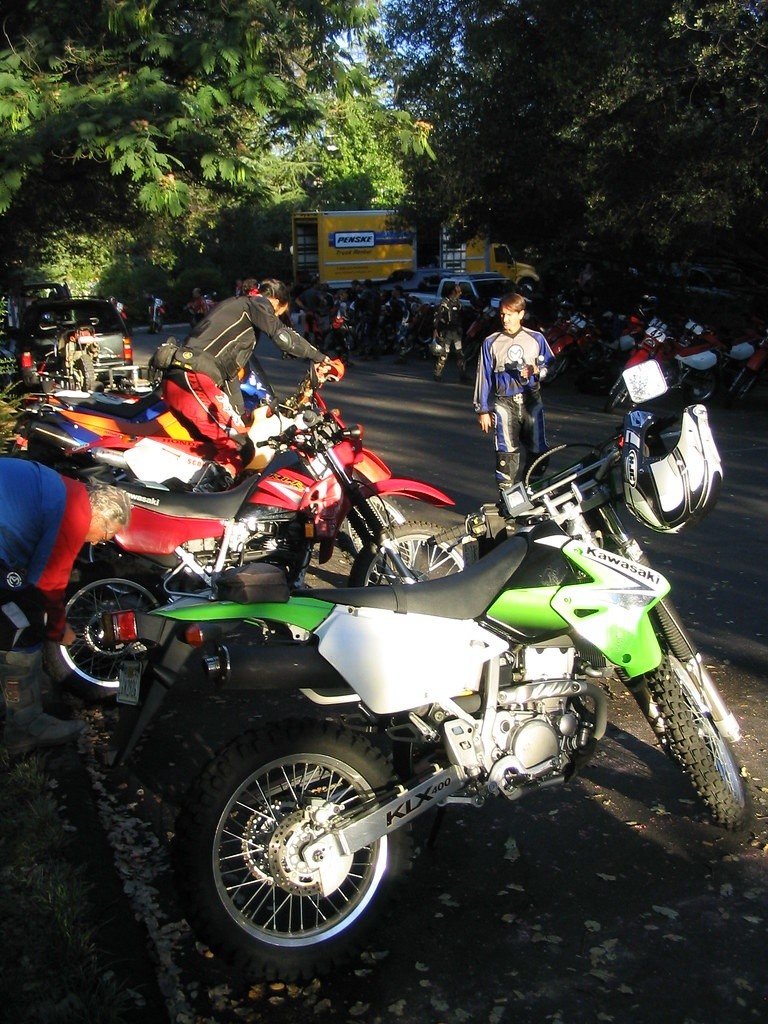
407, 273, 534, 309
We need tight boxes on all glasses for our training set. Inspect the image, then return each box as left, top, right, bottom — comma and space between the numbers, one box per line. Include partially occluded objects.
99, 521, 109, 545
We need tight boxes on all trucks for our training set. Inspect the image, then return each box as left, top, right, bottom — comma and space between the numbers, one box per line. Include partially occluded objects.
290, 210, 539, 303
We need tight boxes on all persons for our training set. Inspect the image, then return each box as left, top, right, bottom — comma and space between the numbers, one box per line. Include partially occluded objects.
235, 279, 243, 295
0, 457, 132, 753
149, 294, 165, 333
295, 272, 467, 382
191, 287, 207, 322
108, 295, 128, 324
473, 291, 556, 496
161, 278, 331, 493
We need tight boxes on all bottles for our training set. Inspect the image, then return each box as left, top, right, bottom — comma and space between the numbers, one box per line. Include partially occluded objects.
517, 359, 529, 385
535, 355, 545, 380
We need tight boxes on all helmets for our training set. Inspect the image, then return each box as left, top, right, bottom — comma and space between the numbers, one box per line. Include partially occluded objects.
428, 336, 447, 356
623, 404, 723, 535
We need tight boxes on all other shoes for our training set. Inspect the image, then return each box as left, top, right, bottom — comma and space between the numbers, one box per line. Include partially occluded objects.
434, 375, 442, 381
461, 375, 471, 382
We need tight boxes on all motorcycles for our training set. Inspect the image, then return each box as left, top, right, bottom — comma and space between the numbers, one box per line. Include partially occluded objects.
9, 292, 768, 986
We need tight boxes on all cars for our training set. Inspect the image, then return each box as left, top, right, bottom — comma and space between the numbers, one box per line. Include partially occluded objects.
685, 267, 767, 301
16, 296, 133, 392
373, 268, 455, 290
